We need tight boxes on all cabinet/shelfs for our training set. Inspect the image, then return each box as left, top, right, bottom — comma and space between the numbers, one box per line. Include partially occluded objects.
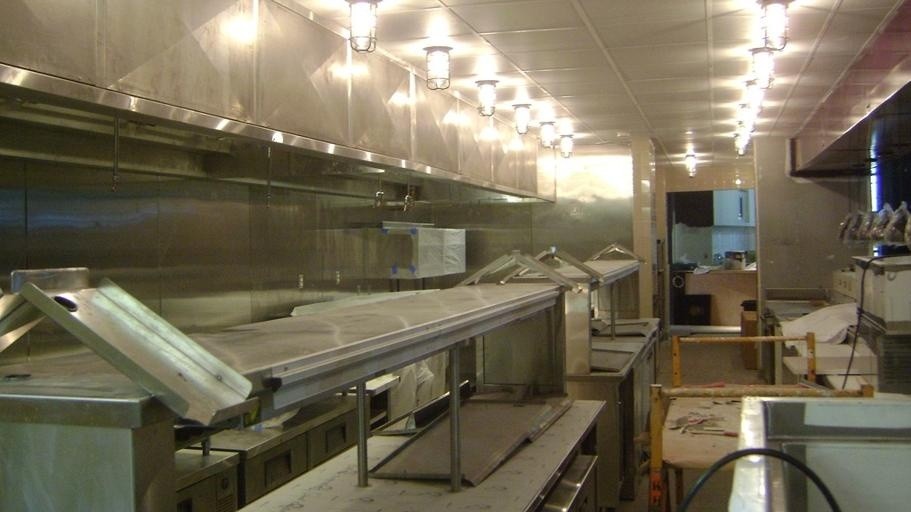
675, 189, 758, 229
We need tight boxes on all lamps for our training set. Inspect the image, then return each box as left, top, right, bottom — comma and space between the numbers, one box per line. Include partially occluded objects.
684, 155, 696, 179
733, 1, 791, 158
